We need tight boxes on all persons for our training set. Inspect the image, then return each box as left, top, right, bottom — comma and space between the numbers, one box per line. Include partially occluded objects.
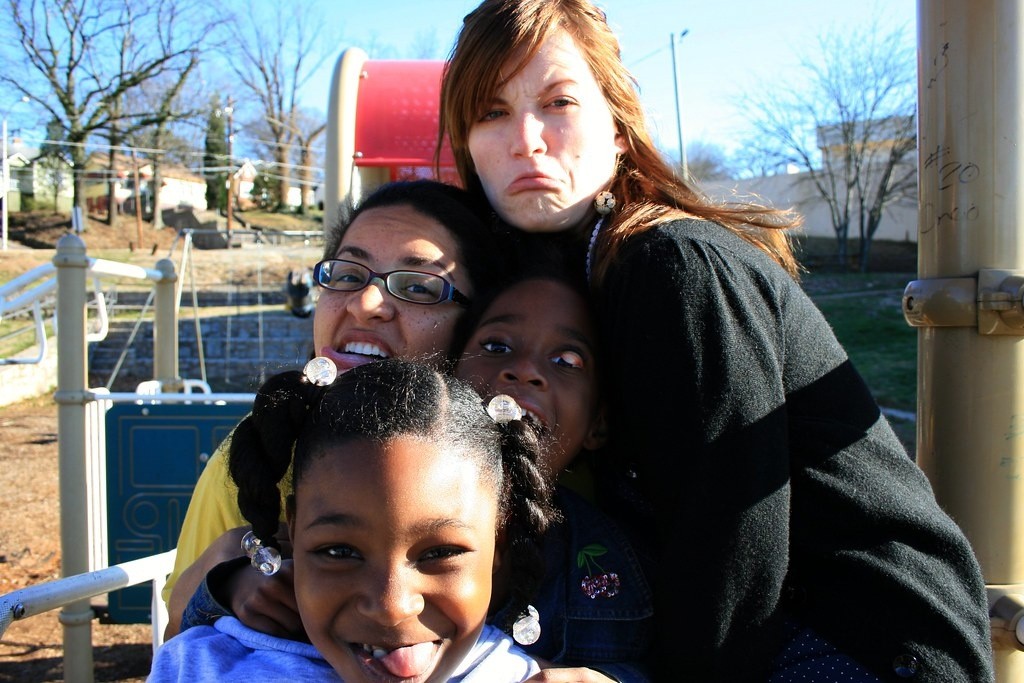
161, 180, 494, 642
148, 357, 556, 683
180, 270, 657, 683
433, 0, 997, 683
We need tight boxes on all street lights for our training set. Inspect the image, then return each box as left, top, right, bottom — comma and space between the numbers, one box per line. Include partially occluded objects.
0, 95, 30, 252
668, 29, 692, 182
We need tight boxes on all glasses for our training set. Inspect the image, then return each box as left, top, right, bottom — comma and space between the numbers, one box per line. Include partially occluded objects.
313, 258, 473, 306
